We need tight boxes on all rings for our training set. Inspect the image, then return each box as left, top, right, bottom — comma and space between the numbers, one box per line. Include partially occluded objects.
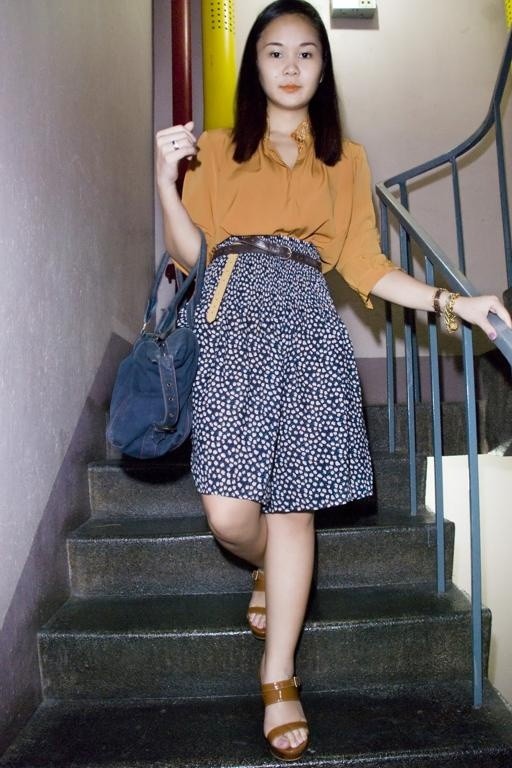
170, 140, 183, 151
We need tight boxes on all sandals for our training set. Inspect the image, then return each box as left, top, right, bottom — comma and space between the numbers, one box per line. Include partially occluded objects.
257, 675, 309, 760
246, 569, 270, 641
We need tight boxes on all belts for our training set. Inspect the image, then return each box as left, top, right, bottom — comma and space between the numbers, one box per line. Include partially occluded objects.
206, 235, 322, 324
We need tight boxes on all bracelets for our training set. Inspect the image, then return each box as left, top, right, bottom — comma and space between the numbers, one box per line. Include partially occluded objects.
442, 291, 461, 333
434, 286, 448, 317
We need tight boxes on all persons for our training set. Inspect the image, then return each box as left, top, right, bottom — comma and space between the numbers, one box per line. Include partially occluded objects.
149, 0, 512, 760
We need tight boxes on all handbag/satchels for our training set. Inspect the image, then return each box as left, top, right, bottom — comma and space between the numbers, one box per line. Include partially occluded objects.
107, 226, 206, 460
